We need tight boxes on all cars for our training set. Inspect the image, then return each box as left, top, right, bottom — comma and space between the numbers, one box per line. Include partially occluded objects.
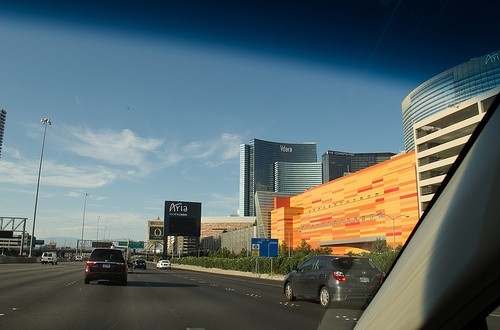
134, 259, 146, 269
156, 260, 171, 270
76, 255, 83, 262
282, 254, 386, 308
127, 258, 136, 268
85, 249, 128, 285
41, 252, 58, 265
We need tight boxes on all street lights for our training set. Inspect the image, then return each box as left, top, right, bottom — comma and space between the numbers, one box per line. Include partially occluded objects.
375, 212, 409, 254
80, 192, 89, 256
27, 118, 53, 259
